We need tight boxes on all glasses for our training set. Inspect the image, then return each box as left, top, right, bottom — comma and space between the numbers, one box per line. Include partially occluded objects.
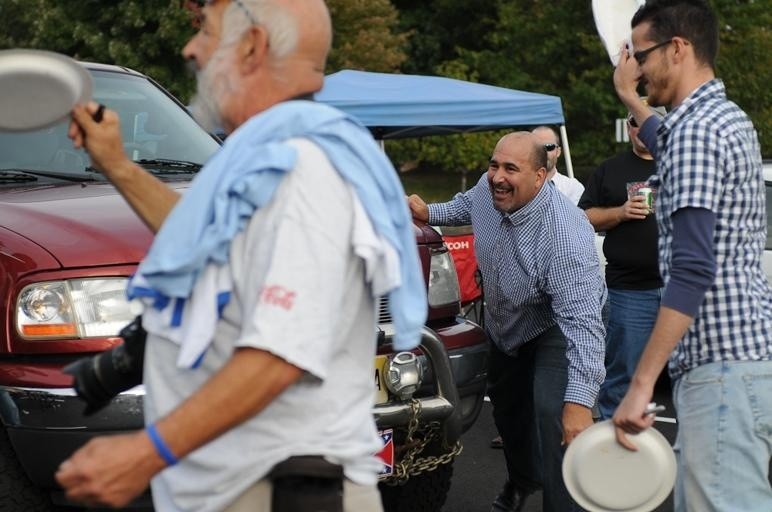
544, 143, 559, 151
633, 40, 689, 66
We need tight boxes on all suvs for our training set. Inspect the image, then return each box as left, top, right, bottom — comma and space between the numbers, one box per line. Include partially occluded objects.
0, 44, 498, 512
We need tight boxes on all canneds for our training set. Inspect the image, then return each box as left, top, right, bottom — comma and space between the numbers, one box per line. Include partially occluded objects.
638, 188, 652, 213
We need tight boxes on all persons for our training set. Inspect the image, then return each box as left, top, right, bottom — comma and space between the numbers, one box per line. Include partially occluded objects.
612, 0, 771, 510
56, 1, 436, 511
527, 124, 585, 207
577, 98, 668, 422
405, 132, 608, 511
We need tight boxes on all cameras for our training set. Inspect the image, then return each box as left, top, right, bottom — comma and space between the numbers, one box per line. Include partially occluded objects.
63, 314, 147, 413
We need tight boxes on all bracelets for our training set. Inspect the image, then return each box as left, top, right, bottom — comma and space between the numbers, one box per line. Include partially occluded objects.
144, 422, 178, 465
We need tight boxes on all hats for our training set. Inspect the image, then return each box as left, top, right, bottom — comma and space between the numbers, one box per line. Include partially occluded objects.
627, 96, 667, 120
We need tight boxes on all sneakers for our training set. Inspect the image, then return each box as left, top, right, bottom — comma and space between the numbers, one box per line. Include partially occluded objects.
492, 482, 527, 511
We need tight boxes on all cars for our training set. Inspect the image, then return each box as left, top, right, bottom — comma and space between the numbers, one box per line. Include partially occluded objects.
760, 157, 772, 281
